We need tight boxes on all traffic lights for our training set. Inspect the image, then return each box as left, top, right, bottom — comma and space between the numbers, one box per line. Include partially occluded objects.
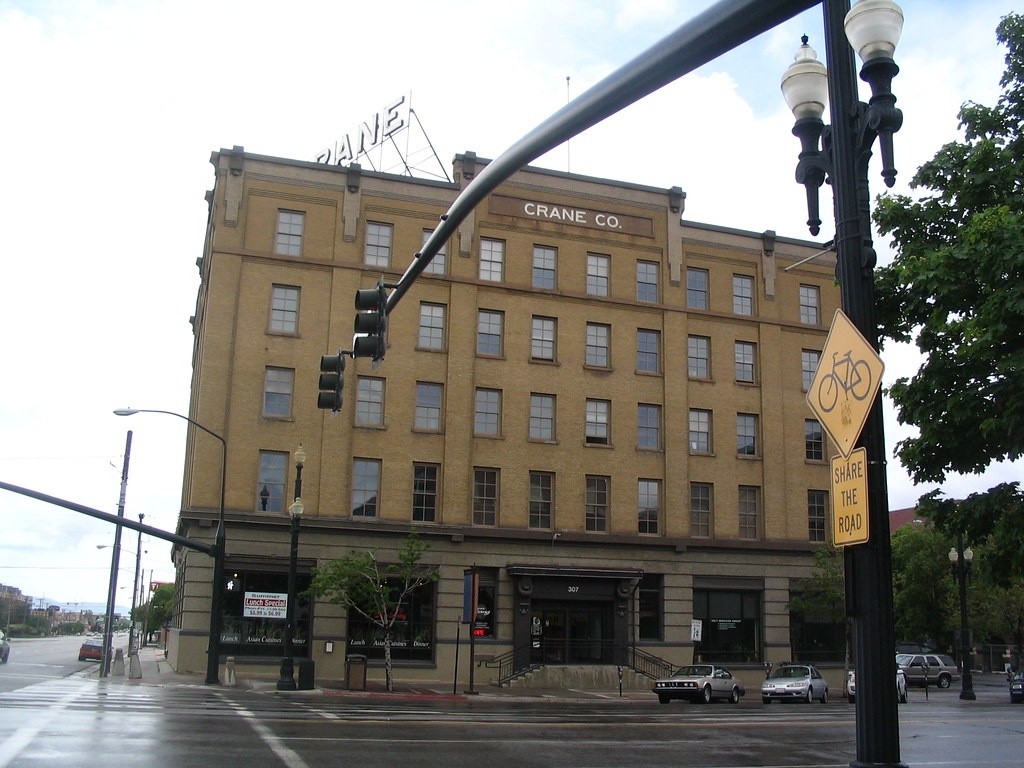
353, 275, 386, 362
318, 354, 346, 412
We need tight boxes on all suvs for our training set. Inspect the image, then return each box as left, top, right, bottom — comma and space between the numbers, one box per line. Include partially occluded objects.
897, 654, 961, 689
847, 662, 908, 703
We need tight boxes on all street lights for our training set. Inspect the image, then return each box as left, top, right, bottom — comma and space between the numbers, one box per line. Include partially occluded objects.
778, 3, 907, 767
277, 444, 306, 691
113, 408, 227, 685
97, 544, 144, 657
948, 546, 977, 700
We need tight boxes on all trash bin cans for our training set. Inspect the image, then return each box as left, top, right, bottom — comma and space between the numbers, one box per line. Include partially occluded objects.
1005, 663, 1011, 673
298, 661, 315, 690
344, 654, 367, 691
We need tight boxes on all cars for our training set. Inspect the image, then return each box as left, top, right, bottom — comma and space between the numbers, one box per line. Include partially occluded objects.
86, 632, 91, 636
1007, 665, 1024, 702
761, 664, 829, 704
653, 664, 745, 704
0, 630, 11, 663
78, 636, 104, 662
